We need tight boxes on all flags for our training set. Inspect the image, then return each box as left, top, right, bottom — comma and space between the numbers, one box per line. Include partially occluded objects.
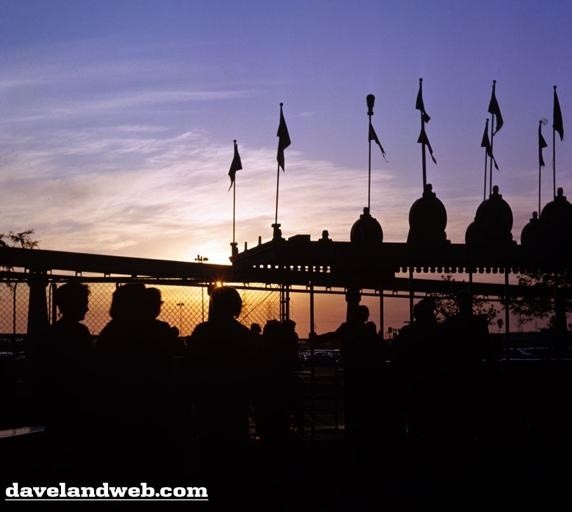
227, 142, 243, 190
538, 124, 548, 167
413, 82, 429, 124
552, 88, 564, 142
275, 111, 291, 171
481, 121, 499, 174
417, 120, 437, 166
367, 122, 388, 161
488, 86, 504, 136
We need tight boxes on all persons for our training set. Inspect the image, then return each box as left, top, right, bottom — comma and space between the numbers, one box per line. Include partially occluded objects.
14, 270, 489, 497
